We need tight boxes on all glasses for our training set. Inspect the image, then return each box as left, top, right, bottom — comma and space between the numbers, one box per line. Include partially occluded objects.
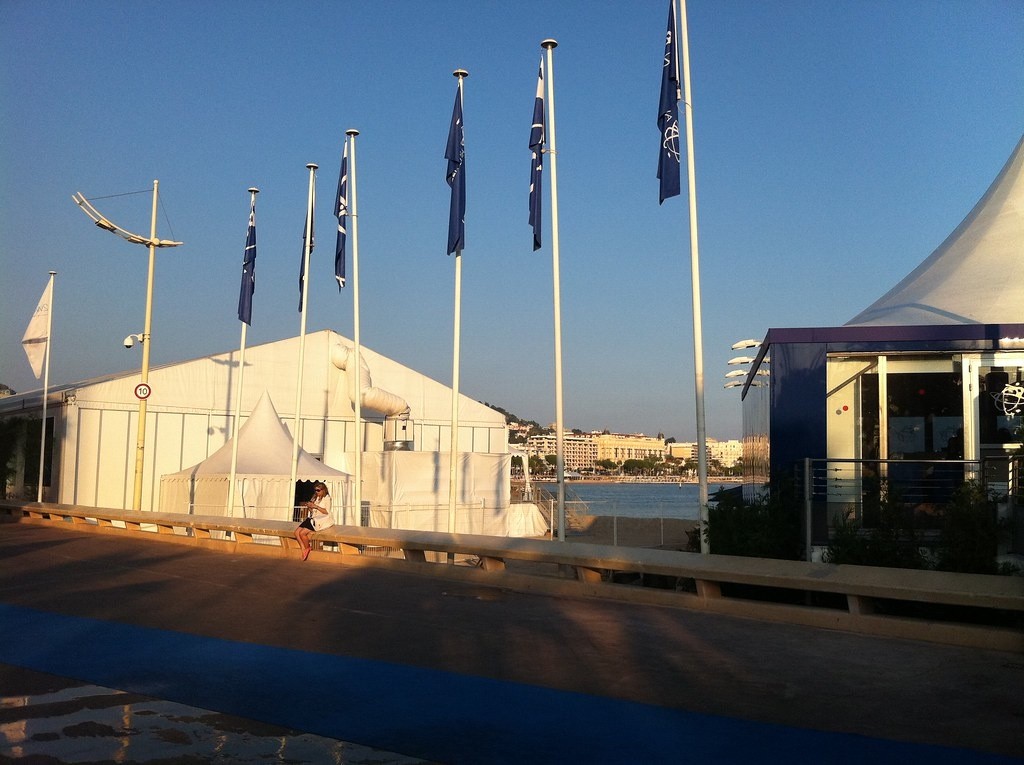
315, 489, 321, 492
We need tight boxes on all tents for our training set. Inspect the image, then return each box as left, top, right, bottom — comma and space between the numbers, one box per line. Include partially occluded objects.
157, 388, 360, 552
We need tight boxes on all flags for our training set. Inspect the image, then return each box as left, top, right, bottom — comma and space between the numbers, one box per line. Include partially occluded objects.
333, 142, 348, 292
22, 278, 51, 379
656, 0, 681, 205
237, 204, 257, 328
528, 55, 546, 252
299, 189, 314, 311
444, 84, 465, 255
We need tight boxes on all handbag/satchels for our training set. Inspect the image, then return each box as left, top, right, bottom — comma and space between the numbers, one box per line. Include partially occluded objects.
310, 513, 335, 531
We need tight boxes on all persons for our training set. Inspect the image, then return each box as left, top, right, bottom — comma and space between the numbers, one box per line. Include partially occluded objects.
295, 482, 335, 561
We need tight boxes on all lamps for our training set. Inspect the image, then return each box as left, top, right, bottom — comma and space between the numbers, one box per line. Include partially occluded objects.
728, 357, 756, 365
731, 339, 762, 351
725, 370, 749, 378
724, 381, 741, 388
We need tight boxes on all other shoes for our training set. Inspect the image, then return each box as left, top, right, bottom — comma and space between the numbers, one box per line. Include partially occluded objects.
302, 547, 311, 560
